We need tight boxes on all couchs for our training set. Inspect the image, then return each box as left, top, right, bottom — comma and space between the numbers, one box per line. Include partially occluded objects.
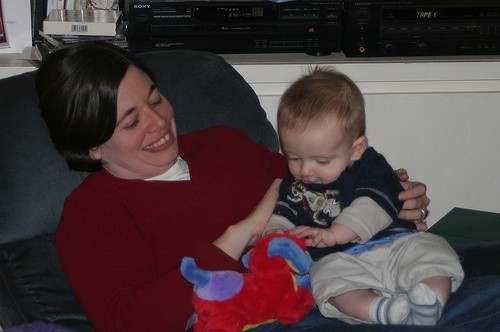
0, 47, 281, 332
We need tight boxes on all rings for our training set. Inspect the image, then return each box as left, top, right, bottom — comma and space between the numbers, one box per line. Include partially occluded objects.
420, 208, 428, 221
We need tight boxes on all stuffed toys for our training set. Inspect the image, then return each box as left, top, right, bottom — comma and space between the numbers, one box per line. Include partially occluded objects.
181, 229, 313, 331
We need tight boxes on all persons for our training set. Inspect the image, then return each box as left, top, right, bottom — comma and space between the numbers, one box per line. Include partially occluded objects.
31, 40, 500, 332
250, 65, 464, 325
